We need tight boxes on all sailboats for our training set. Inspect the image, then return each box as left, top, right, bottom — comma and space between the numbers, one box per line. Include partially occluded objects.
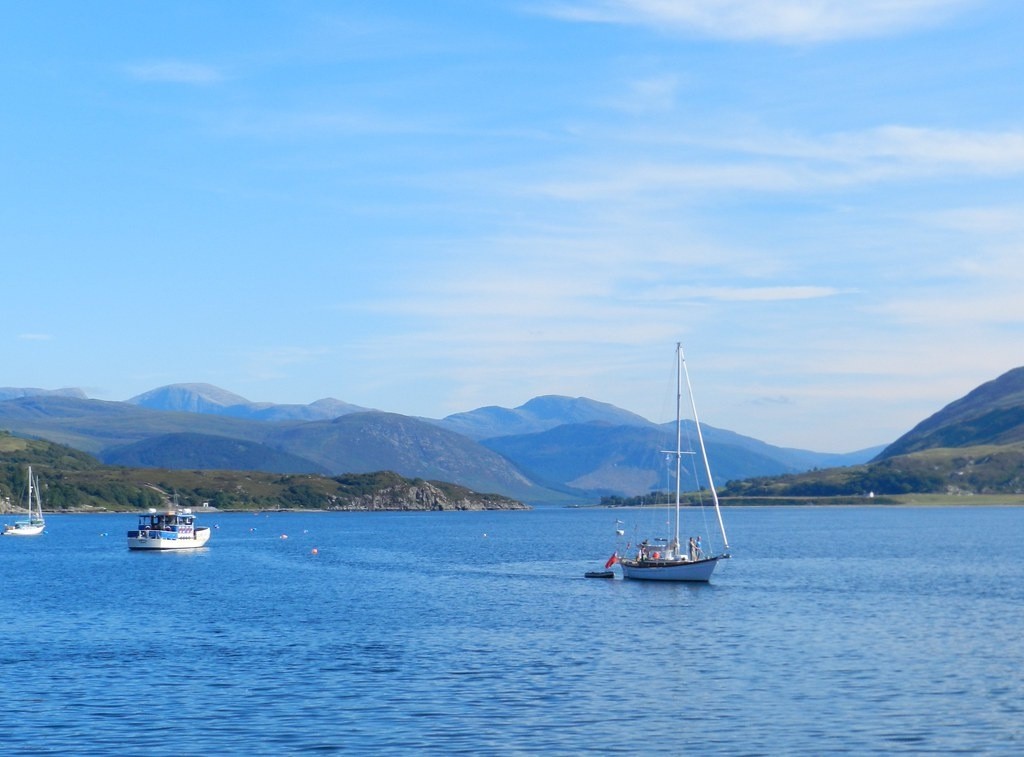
14, 476, 43, 527
610, 341, 732, 584
5, 466, 45, 535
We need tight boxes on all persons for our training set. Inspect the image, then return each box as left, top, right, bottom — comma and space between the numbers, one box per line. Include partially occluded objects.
639, 547, 646, 560
689, 536, 704, 560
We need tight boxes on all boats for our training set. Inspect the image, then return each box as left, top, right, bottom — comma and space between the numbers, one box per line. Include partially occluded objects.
584, 571, 614, 578
127, 510, 211, 550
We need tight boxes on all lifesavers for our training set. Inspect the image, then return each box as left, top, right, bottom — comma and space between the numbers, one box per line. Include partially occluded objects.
179, 525, 192, 534
144, 525, 151, 532
585, 571, 614, 578
165, 526, 171, 531
